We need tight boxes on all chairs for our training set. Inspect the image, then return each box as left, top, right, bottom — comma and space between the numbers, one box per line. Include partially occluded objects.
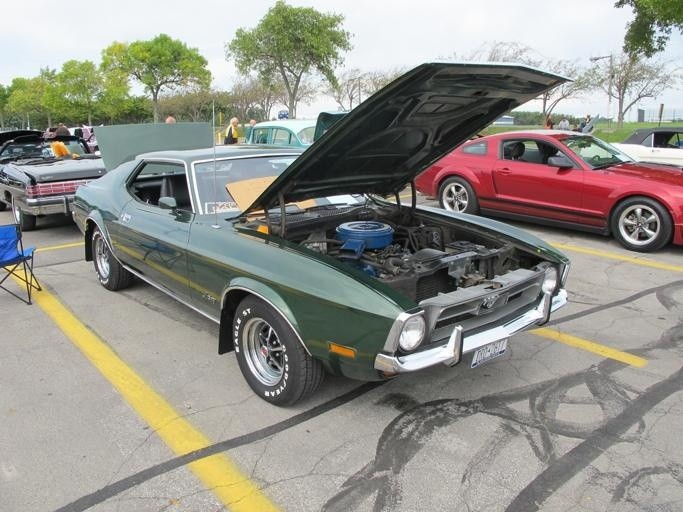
0, 224, 42, 305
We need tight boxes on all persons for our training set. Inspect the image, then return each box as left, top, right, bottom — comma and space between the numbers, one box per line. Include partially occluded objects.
558, 117, 569, 129
223, 118, 239, 144
546, 115, 556, 129
50, 141, 70, 157
244, 120, 256, 142
584, 115, 593, 130
165, 116, 175, 123
55, 123, 71, 136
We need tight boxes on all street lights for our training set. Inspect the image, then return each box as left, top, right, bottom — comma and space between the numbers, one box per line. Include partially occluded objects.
589, 55, 613, 124
